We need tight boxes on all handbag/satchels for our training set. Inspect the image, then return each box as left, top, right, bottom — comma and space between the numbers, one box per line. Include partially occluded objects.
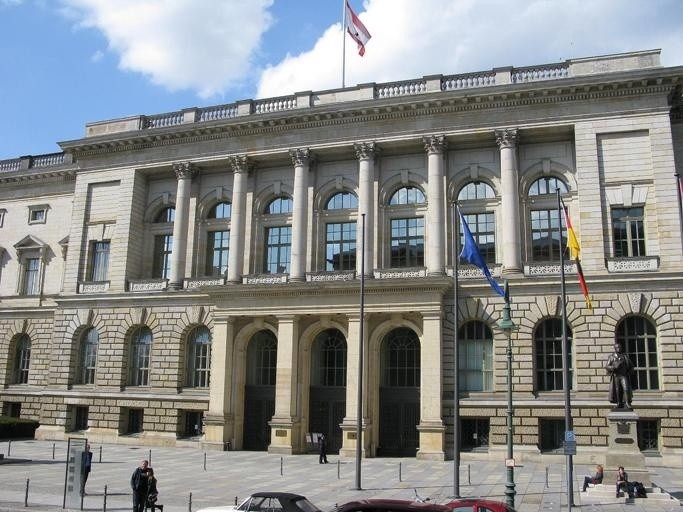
148, 492, 157, 502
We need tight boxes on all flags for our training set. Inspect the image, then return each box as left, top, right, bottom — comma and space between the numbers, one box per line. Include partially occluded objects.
456, 205, 508, 300
345, 1, 372, 57
558, 197, 594, 311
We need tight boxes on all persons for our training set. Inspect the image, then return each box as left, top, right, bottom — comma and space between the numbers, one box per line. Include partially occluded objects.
145, 467, 164, 512
616, 465, 634, 498
606, 342, 636, 410
79, 444, 93, 497
318, 434, 329, 464
581, 465, 604, 492
129, 459, 148, 512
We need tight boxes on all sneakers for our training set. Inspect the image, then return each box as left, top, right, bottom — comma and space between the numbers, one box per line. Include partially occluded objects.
160, 505, 163, 512
